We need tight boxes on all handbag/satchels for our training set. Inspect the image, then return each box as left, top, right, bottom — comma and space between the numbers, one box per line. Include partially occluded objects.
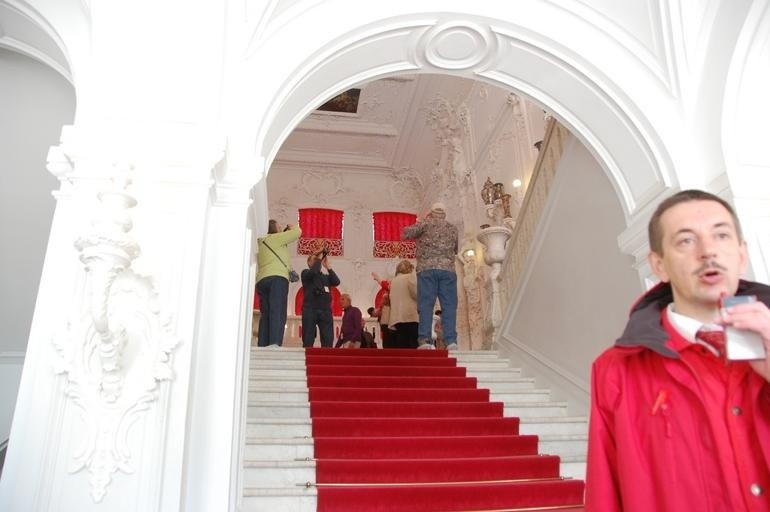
290, 270, 300, 283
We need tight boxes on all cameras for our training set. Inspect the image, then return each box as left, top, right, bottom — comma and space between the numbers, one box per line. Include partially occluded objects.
314, 248, 329, 260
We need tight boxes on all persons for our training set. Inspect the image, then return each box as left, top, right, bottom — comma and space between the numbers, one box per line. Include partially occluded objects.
400, 203, 459, 350
583, 190, 770, 512
257, 219, 303, 347
334, 261, 442, 349
301, 251, 340, 347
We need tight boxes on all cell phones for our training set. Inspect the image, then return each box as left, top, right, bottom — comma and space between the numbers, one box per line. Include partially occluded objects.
284, 227, 288, 232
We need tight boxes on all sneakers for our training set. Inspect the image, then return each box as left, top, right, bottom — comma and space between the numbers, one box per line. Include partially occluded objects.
416, 342, 459, 351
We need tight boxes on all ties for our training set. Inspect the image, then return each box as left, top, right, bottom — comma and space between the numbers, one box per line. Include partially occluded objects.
697, 330, 727, 361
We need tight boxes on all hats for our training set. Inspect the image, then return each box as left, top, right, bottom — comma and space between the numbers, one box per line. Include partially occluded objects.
430, 202, 448, 214
